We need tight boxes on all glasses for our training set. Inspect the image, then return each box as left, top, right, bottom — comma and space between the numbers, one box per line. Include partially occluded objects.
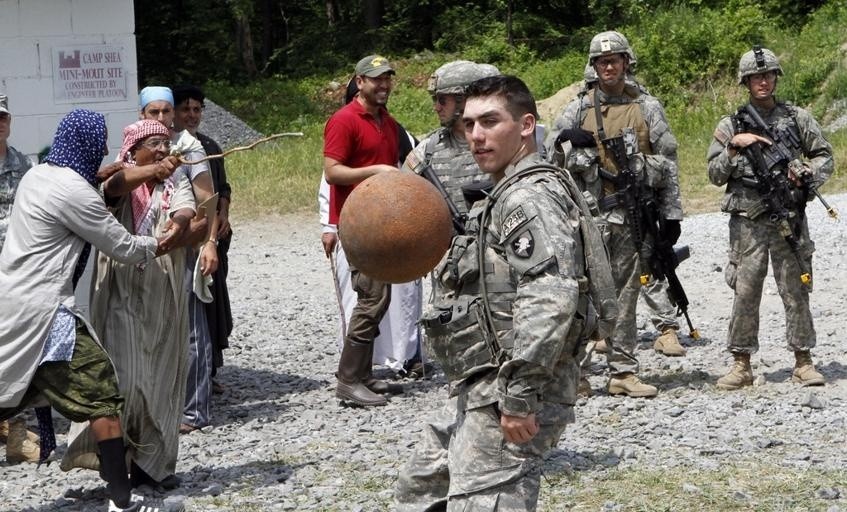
749, 71, 777, 83
432, 94, 447, 105
594, 54, 624, 66
142, 138, 171, 148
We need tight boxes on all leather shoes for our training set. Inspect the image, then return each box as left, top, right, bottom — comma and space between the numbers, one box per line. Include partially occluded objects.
212, 381, 225, 394
335, 382, 388, 406
360, 380, 404, 395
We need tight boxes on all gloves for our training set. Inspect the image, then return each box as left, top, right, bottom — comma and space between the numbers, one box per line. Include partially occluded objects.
659, 220, 681, 245
560, 127, 597, 149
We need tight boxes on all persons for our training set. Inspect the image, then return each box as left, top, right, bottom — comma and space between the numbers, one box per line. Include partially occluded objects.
1, 110, 187, 512
165, 81, 233, 396
705, 44, 839, 393
402, 60, 546, 224
0, 95, 33, 253
318, 54, 431, 409
553, 31, 688, 397
413, 75, 623, 512
115, 86, 218, 435
89, 116, 194, 498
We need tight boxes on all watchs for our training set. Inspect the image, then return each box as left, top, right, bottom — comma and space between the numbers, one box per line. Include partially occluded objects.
208, 236, 221, 247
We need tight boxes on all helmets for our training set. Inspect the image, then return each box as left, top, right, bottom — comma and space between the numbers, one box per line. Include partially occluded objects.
427, 59, 483, 95
478, 62, 501, 77
737, 44, 785, 86
583, 62, 599, 84
588, 29, 634, 66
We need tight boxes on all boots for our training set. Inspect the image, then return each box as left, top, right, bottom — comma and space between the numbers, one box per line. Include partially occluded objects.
6, 420, 56, 464
715, 353, 753, 389
654, 326, 687, 356
0, 418, 41, 444
792, 350, 826, 387
607, 372, 658, 398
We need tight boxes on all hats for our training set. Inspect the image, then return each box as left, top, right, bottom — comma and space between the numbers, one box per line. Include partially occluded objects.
0, 94, 10, 114
354, 54, 396, 79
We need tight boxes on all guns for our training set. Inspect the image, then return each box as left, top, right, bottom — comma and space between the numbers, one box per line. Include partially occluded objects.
598, 135, 650, 284
651, 223, 701, 340
741, 104, 837, 217
741, 144, 809, 283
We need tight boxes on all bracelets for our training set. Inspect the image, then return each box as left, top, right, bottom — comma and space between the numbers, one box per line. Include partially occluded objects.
727, 140, 742, 153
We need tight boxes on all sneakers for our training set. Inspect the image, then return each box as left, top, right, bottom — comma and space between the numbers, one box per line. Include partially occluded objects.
107, 488, 185, 512
